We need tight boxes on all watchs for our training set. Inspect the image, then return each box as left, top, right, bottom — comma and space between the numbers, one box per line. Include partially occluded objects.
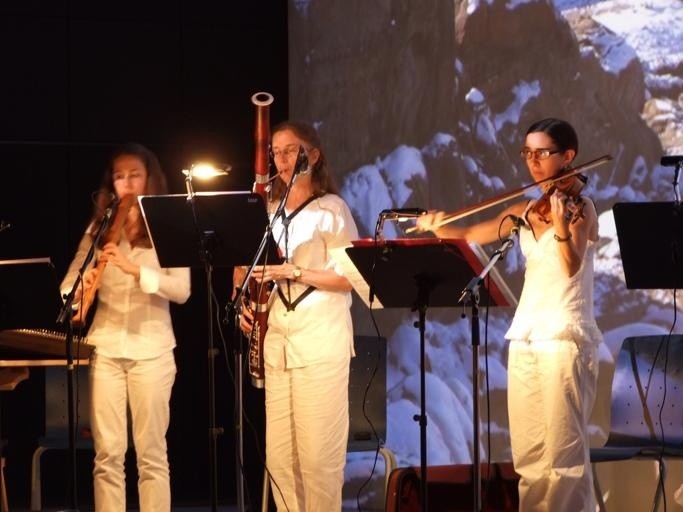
292, 264, 302, 284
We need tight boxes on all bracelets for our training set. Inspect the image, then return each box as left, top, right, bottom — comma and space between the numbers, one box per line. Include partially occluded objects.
551, 233, 573, 242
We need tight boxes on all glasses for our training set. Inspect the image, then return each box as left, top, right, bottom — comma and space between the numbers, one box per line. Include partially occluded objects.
520, 148, 565, 160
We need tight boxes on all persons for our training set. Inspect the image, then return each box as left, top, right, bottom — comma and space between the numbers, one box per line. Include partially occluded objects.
56, 140, 192, 512
414, 116, 606, 512
229, 122, 360, 512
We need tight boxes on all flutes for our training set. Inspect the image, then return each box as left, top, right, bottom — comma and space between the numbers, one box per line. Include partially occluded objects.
73, 192, 133, 327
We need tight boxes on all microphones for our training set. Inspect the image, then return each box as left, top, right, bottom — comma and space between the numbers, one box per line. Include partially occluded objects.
510, 214, 530, 231
659, 155, 683, 167
102, 192, 121, 220
380, 207, 428, 219
299, 144, 310, 174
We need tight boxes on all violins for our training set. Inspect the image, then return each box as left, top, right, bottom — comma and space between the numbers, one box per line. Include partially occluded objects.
529, 164, 588, 226
